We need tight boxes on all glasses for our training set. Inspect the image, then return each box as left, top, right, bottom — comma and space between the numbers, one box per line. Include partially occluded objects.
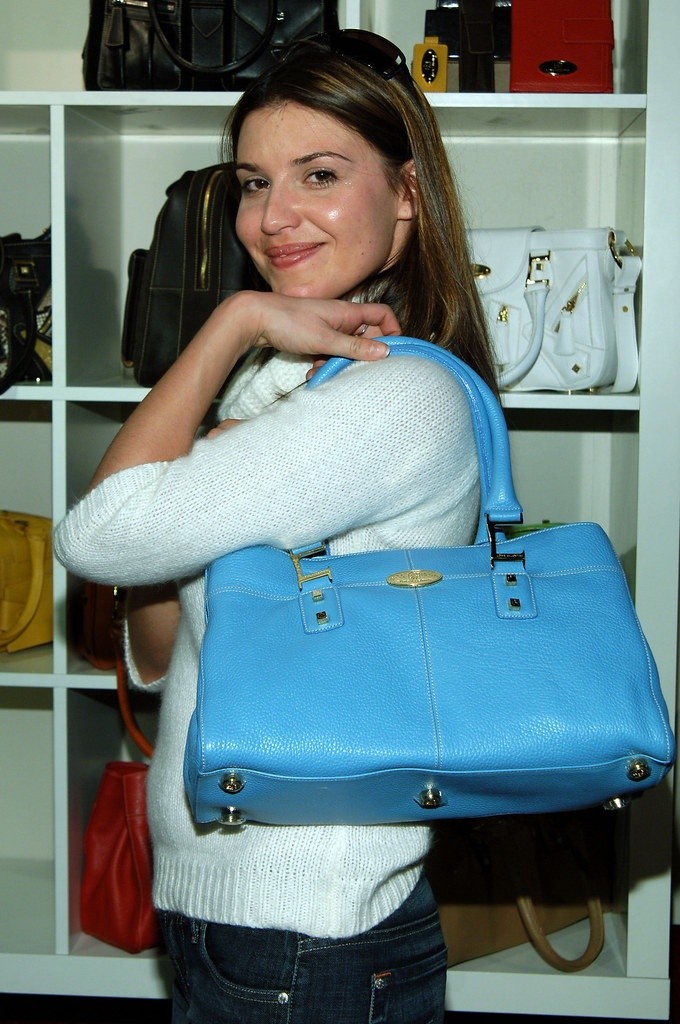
297, 29, 414, 94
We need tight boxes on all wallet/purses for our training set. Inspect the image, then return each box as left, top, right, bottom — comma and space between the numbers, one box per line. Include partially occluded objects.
509, 0, 615, 93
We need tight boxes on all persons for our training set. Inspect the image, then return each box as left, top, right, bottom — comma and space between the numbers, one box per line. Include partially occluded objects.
48, 30, 504, 1024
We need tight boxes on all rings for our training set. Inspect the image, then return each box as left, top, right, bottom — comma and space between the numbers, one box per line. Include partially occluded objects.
356, 324, 369, 336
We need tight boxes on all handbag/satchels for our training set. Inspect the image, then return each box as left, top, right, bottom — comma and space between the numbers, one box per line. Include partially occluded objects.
0, 228, 52, 393
80, 761, 159, 955
425, 809, 628, 972
425, 0, 510, 61
0, 510, 53, 653
68, 579, 118, 670
82, 0, 342, 91
183, 336, 676, 827
453, 226, 641, 393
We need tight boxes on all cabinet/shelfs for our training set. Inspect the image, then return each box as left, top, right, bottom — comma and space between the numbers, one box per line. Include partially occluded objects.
0, 0, 679, 1021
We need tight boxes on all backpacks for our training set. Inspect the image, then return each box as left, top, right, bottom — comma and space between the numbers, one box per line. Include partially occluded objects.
121, 161, 272, 389
